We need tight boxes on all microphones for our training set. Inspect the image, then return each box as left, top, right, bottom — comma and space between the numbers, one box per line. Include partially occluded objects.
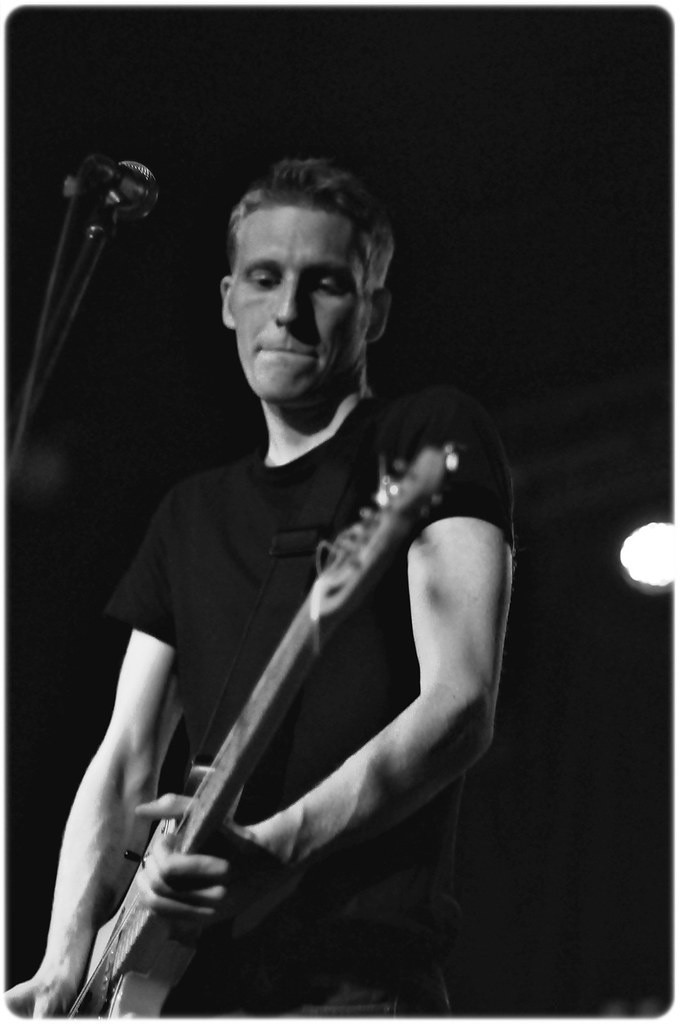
82, 154, 160, 219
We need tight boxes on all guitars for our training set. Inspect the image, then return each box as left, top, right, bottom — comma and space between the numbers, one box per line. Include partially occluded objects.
58, 443, 470, 1018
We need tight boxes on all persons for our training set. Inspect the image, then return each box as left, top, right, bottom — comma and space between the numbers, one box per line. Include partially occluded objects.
6, 151, 517, 1019
